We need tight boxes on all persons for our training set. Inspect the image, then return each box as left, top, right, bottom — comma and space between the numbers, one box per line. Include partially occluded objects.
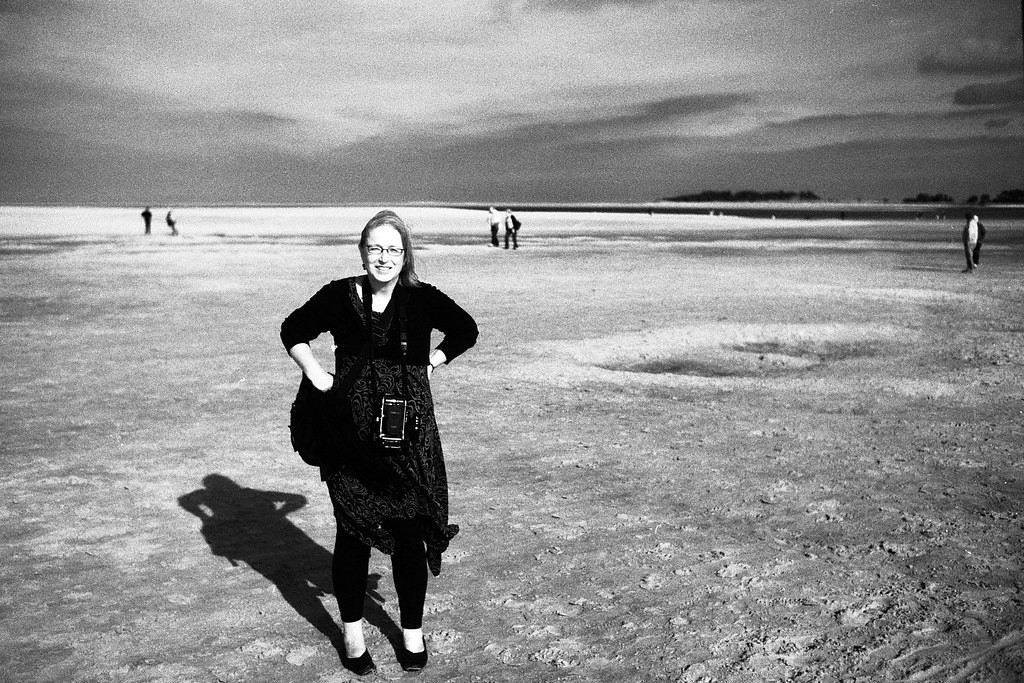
503, 209, 520, 250
280, 211, 478, 677
166, 212, 179, 237
141, 207, 153, 235
486, 206, 502, 247
961, 214, 987, 273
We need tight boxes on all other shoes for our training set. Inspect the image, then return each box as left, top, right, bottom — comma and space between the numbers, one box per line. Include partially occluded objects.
345, 646, 378, 676
403, 636, 428, 672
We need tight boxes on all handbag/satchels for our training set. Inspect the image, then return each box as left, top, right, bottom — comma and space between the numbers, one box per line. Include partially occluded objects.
289, 372, 356, 466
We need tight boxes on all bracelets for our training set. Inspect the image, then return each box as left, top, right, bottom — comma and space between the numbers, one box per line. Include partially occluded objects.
428, 363, 435, 373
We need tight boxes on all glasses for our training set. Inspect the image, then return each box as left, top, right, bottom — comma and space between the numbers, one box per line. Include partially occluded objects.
362, 243, 405, 257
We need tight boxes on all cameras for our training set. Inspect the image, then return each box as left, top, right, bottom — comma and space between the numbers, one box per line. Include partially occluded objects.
380, 397, 407, 440
